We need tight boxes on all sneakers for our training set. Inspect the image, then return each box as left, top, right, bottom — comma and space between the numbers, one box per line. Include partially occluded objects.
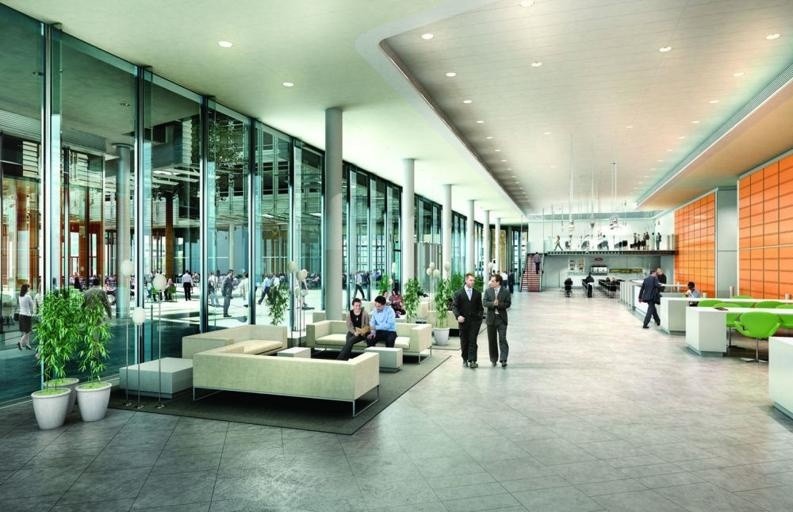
643, 319, 660, 329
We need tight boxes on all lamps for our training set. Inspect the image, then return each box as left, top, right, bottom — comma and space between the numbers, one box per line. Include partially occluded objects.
288, 260, 307, 347
121, 259, 132, 407
427, 262, 450, 311
133, 307, 145, 409
153, 274, 166, 409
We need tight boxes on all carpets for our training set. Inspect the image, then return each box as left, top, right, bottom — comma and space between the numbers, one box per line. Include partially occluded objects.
429, 319, 487, 350
108, 351, 452, 436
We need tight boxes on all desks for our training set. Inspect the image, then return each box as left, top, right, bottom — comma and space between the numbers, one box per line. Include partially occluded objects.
686, 306, 793, 355
768, 336, 793, 419
659, 297, 793, 334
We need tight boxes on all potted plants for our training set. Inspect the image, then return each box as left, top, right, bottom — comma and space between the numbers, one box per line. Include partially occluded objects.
433, 279, 451, 346
30, 287, 112, 430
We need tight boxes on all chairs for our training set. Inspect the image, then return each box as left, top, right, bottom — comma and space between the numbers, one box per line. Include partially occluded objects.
599, 277, 623, 298
697, 295, 793, 363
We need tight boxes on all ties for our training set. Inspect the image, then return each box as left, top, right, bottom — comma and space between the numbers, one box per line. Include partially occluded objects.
495, 289, 499, 312
467, 289, 472, 301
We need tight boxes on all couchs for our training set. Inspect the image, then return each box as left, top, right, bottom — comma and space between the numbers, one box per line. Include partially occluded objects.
192, 344, 379, 417
306, 320, 432, 365
418, 301, 461, 337
182, 324, 287, 362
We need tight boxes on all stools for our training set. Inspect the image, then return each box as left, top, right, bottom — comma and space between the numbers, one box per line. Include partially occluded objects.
277, 346, 311, 358
365, 346, 403, 373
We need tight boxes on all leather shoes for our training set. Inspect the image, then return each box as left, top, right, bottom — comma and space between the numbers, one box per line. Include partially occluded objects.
462, 360, 477, 368
492, 361, 507, 367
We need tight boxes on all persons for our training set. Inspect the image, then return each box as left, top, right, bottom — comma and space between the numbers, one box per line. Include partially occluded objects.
533, 252, 540, 274
483, 276, 511, 367
553, 232, 661, 252
586, 272, 594, 297
491, 269, 515, 294
657, 267, 666, 292
16, 268, 405, 360
639, 270, 663, 328
684, 281, 699, 306
452, 274, 484, 368
